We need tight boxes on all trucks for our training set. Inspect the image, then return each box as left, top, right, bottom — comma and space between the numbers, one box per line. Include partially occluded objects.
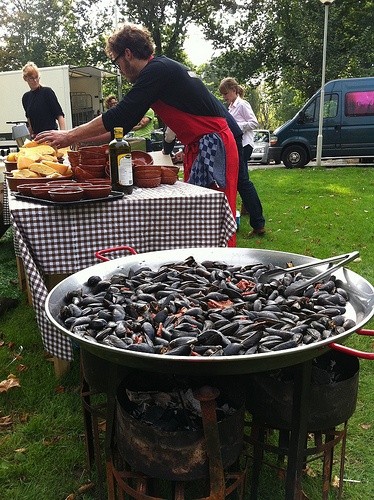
0, 64, 120, 154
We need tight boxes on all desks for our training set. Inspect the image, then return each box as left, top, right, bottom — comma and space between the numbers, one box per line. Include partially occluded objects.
2, 169, 238, 380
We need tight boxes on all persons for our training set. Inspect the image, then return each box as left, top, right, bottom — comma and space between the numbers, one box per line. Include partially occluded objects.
131, 107, 155, 152
34, 25, 245, 249
218, 77, 265, 239
104, 92, 118, 111
20, 61, 65, 135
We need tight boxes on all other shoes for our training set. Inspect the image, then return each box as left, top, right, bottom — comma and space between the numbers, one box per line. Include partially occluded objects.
247, 227, 265, 237
241, 203, 248, 214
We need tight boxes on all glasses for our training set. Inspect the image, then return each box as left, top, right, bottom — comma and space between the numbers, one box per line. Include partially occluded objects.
111, 53, 123, 69
28, 76, 41, 82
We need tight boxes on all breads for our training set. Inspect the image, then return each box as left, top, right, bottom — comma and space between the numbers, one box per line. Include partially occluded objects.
7, 140, 72, 178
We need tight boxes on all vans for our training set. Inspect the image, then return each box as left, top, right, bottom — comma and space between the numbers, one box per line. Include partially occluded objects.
266, 76, 374, 167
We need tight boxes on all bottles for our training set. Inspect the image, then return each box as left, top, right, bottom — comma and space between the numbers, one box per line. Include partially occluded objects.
108, 127, 134, 195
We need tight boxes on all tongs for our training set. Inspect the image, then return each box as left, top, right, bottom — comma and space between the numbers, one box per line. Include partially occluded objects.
257, 250, 360, 296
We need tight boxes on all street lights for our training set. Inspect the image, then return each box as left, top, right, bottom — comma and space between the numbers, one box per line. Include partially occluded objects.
315, 0, 336, 167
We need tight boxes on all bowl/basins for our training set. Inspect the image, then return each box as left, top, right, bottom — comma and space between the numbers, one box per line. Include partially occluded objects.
3, 144, 179, 202
43, 245, 374, 376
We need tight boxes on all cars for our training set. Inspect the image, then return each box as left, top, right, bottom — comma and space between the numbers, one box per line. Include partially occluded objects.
246, 129, 273, 165
140, 127, 185, 152
